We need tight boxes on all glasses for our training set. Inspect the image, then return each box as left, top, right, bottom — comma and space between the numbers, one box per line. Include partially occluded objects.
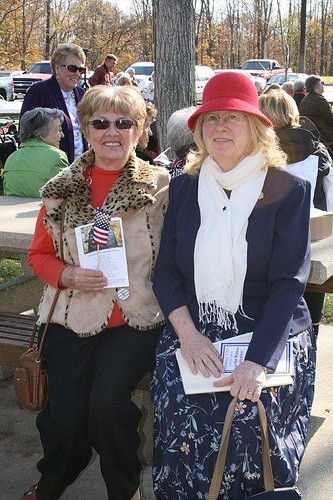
87, 118, 138, 129
49, 127, 63, 133
61, 64, 86, 74
321, 82, 324, 84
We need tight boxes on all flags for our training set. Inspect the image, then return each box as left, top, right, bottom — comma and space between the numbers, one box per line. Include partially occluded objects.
93, 208, 111, 245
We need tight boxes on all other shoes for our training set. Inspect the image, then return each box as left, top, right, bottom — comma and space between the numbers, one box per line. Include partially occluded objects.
19, 484, 37, 500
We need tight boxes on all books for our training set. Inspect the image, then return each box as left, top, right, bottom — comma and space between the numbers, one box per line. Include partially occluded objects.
74, 217, 130, 288
175, 329, 294, 395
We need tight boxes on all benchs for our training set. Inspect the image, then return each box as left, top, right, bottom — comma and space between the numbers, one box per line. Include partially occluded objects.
0, 310, 155, 398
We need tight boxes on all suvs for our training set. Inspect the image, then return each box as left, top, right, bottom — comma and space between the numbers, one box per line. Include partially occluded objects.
12, 60, 94, 95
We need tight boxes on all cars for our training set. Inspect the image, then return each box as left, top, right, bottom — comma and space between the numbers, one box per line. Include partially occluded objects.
262, 72, 310, 93
137, 65, 217, 105
236, 58, 282, 90
128, 61, 155, 84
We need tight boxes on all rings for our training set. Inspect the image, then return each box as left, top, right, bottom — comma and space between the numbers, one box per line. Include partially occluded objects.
247, 391, 254, 395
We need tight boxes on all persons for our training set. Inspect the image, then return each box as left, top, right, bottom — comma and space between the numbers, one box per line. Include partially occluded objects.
3, 107, 70, 198
89, 54, 117, 87
258, 89, 330, 211
151, 79, 317, 500
113, 67, 139, 86
135, 99, 202, 179
300, 76, 333, 154
20, 84, 170, 500
18, 43, 91, 164
255, 80, 309, 111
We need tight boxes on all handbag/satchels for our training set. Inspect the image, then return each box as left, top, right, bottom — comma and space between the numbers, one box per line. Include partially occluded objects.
11, 347, 48, 409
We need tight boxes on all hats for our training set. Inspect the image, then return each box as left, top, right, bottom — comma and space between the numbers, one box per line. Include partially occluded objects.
188, 72, 273, 130
